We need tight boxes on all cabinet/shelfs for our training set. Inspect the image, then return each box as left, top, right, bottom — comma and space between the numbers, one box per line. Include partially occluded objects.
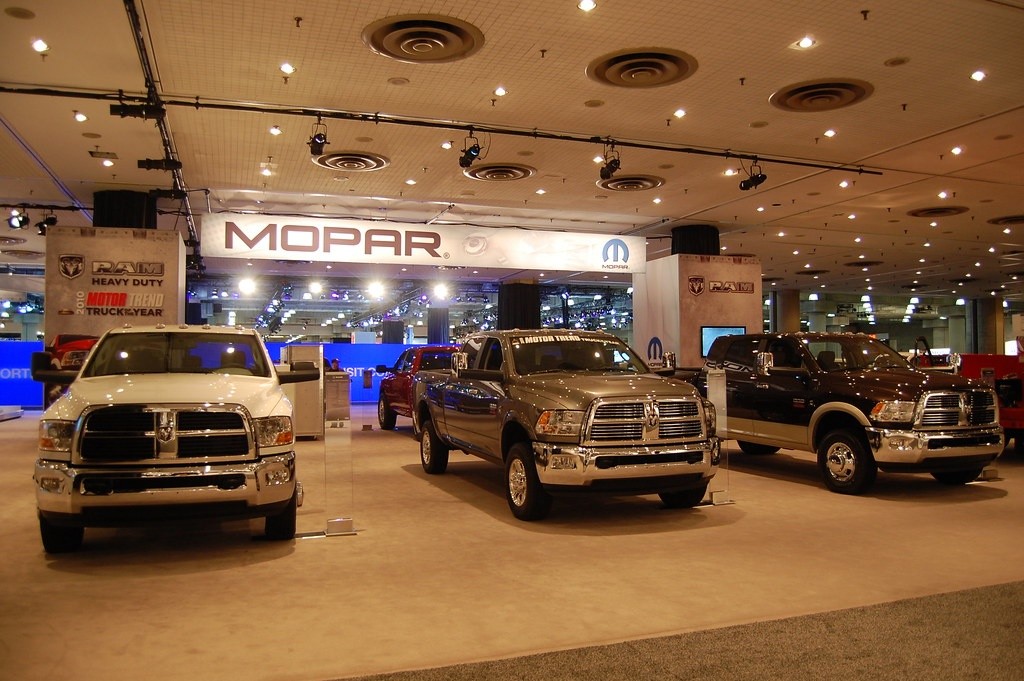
280, 344, 325, 440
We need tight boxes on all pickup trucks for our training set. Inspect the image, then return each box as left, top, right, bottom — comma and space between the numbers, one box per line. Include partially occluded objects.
413, 328, 721, 522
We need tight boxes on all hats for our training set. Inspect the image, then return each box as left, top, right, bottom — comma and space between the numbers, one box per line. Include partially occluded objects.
331, 358, 340, 363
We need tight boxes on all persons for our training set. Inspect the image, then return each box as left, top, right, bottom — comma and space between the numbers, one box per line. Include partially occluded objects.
327, 358, 353, 382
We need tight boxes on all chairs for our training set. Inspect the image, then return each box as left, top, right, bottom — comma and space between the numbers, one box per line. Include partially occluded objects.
818, 351, 836, 370
220, 350, 247, 368
538, 354, 558, 370
128, 351, 158, 371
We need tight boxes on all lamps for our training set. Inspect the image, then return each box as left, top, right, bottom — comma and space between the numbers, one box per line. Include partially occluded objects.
109, 104, 166, 118
149, 187, 187, 199
459, 147, 480, 167
310, 134, 326, 155
8, 212, 30, 229
599, 159, 621, 179
740, 173, 767, 191
35, 213, 57, 236
136, 158, 184, 171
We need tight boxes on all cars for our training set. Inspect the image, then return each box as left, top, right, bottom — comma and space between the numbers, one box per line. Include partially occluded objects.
50, 334, 101, 366
376, 346, 462, 430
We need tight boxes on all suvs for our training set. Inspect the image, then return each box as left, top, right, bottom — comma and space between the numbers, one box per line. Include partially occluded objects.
32, 322, 320, 554
667, 331, 1004, 494
